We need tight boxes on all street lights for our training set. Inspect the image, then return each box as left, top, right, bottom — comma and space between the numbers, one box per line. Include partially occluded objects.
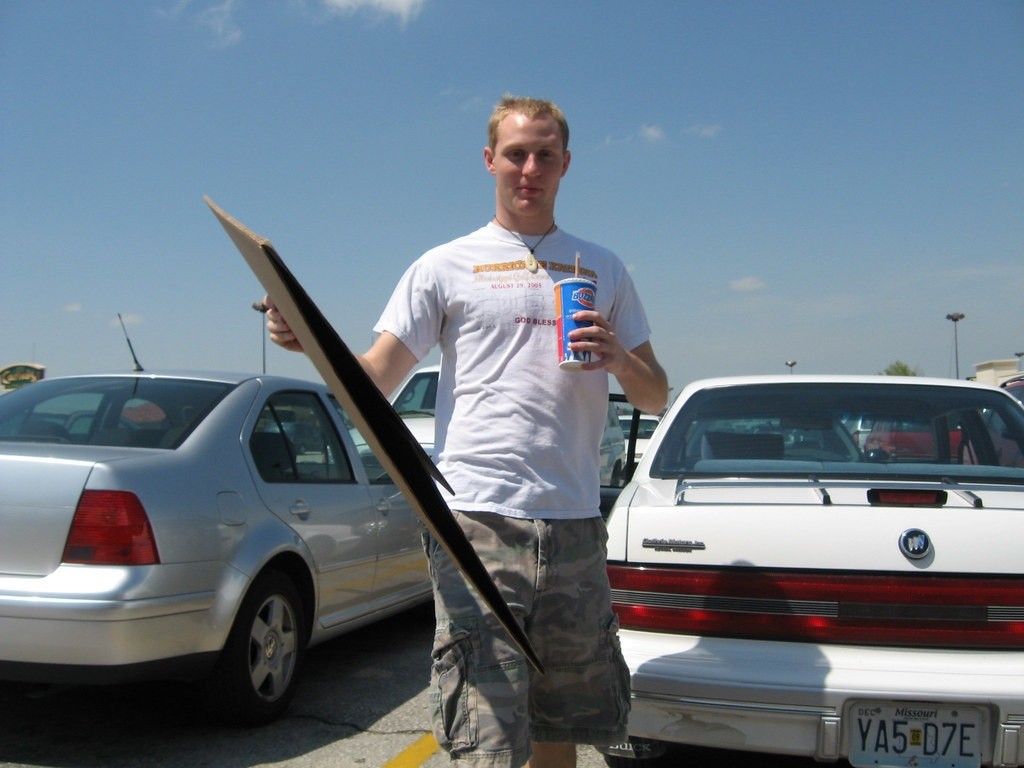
946, 312, 965, 379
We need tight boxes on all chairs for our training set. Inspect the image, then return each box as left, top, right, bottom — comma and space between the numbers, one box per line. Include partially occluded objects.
251, 432, 295, 481
23, 419, 71, 444
91, 429, 144, 447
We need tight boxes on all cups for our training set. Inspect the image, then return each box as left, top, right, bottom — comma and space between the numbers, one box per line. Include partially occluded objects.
553, 278, 597, 372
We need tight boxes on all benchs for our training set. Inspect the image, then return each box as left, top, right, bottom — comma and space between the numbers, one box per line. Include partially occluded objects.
694, 457, 1024, 479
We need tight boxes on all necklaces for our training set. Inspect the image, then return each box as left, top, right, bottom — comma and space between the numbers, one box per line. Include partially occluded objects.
494, 215, 555, 273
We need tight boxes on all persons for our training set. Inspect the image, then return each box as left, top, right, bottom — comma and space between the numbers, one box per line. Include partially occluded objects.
263, 92, 669, 768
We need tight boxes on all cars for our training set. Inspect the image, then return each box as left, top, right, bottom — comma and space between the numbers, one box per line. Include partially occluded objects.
617, 376, 1024, 465
0, 364, 626, 726
606, 374, 1024, 768
